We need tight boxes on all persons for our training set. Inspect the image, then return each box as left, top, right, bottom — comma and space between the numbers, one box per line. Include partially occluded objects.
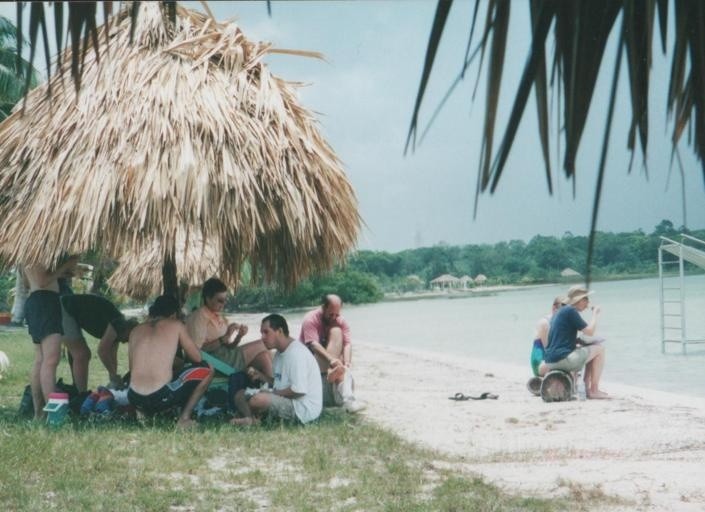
530, 294, 569, 399
544, 284, 612, 399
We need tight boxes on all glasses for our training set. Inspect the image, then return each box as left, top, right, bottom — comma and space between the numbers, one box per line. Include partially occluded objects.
214, 297, 226, 304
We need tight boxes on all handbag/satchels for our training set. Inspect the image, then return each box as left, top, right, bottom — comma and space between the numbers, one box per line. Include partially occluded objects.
17, 370, 131, 421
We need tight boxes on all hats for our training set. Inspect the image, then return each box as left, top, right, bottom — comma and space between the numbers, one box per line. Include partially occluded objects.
561, 285, 594, 306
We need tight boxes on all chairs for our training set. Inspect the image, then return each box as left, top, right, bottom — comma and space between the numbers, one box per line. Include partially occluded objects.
182, 349, 235, 417
